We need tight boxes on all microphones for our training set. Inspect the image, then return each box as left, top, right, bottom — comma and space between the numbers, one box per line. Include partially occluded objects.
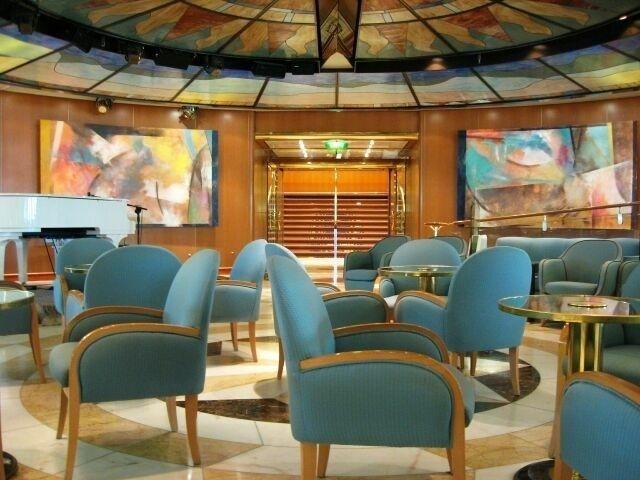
126, 203, 148, 212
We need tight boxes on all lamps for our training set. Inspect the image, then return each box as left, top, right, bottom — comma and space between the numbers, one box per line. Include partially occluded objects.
95, 96, 114, 114
181, 106, 199, 119
